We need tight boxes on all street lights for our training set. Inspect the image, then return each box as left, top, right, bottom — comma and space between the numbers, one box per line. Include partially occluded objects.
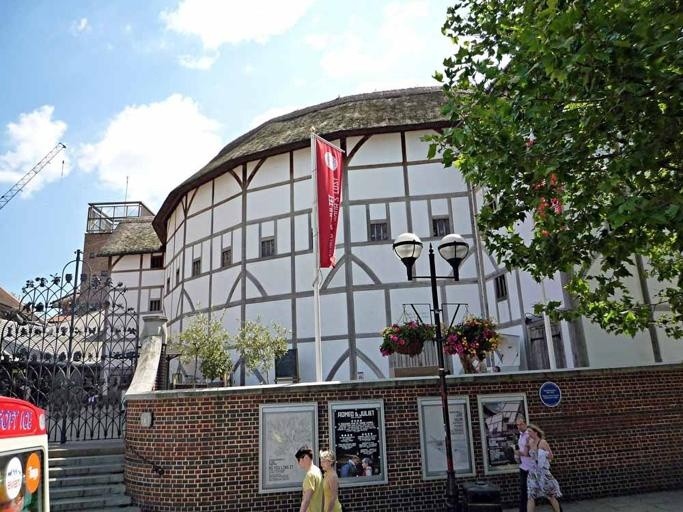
392, 230, 470, 511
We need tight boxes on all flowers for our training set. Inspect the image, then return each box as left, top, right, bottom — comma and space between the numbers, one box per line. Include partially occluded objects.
380, 303, 437, 358
440, 305, 505, 358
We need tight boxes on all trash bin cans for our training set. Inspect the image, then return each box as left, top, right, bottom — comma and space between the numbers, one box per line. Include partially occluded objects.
463, 481, 502, 512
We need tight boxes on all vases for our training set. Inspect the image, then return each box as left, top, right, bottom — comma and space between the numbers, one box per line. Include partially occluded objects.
394, 338, 425, 356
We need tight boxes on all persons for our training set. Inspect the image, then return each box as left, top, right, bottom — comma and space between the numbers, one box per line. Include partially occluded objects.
340, 455, 373, 477
514, 412, 563, 512
295, 444, 342, 512
